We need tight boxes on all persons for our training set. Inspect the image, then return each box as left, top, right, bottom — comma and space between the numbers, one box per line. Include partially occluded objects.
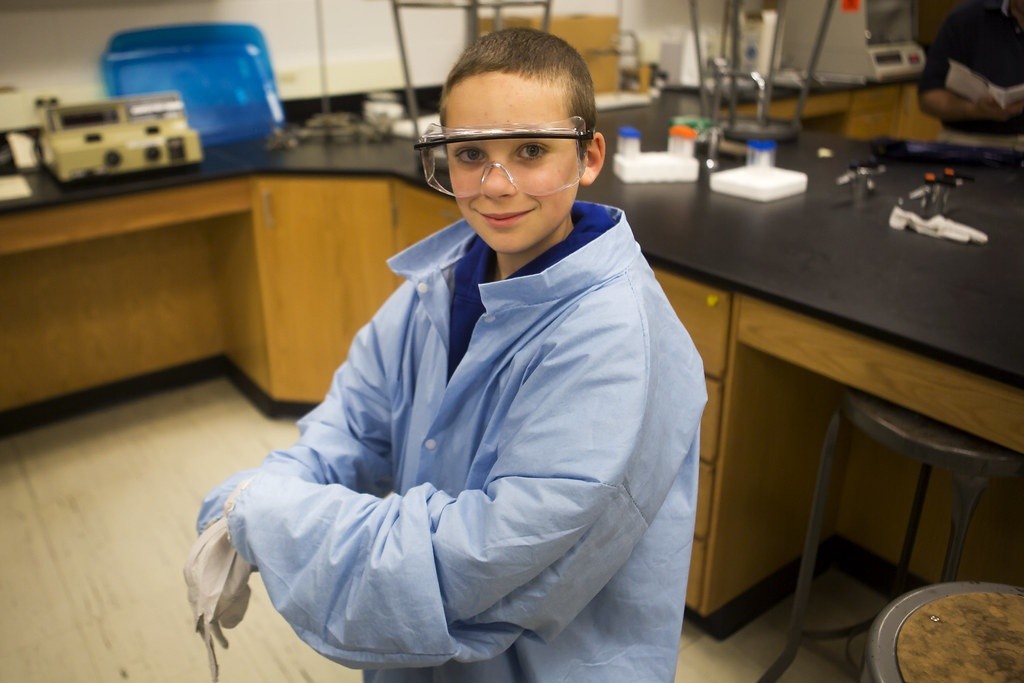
196, 30, 708, 683
918, 0, 1024, 151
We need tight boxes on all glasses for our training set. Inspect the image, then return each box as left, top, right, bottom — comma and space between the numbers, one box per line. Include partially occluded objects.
413, 116, 595, 199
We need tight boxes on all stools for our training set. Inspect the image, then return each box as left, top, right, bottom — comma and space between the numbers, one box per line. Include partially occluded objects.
757, 389, 1024, 683
861, 582, 1024, 683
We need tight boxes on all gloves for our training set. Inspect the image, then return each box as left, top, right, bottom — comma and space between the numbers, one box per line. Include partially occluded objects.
183, 516, 253, 682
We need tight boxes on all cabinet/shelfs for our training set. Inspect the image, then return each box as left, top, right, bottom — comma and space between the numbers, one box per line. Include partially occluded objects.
722, 87, 944, 141
0, 178, 1024, 683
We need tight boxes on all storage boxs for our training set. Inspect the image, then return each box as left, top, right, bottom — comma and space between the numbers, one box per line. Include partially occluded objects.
477, 14, 622, 102
104, 23, 280, 150
